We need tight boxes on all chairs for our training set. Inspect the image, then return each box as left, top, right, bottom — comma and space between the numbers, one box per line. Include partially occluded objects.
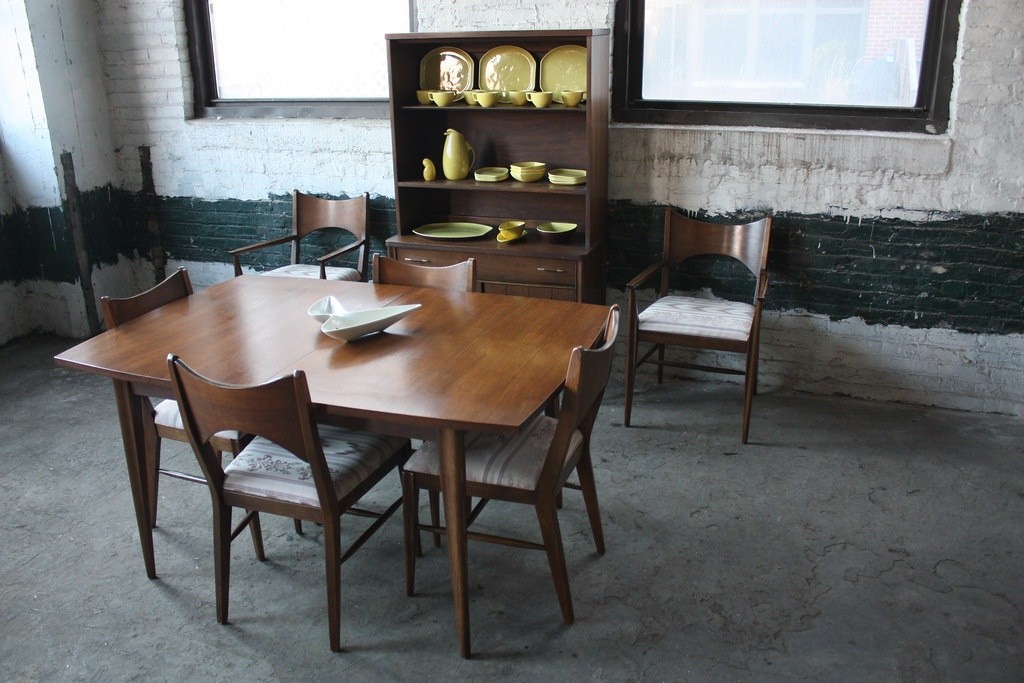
371, 252, 479, 289
624, 205, 773, 444
99, 266, 302, 562
164, 354, 424, 653
396, 303, 622, 624
228, 189, 370, 283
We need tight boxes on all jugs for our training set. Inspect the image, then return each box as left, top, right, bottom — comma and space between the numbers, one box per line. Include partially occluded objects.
442, 128, 475, 180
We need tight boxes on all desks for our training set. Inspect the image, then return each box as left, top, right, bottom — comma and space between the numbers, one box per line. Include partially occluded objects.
52, 273, 613, 657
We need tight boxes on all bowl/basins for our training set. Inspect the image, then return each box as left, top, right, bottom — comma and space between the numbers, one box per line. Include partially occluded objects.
536, 222, 578, 243
510, 160, 546, 183
498, 220, 525, 239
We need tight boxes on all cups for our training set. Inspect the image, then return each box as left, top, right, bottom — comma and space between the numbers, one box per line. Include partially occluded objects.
416, 88, 553, 108
561, 89, 583, 106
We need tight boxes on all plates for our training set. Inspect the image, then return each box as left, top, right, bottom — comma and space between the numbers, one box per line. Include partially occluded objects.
547, 168, 586, 185
475, 167, 509, 181
496, 229, 528, 243
478, 46, 536, 103
539, 45, 587, 103
411, 222, 493, 238
419, 46, 474, 102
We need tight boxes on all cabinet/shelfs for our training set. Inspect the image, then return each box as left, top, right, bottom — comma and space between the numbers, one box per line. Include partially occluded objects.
384, 26, 611, 305
